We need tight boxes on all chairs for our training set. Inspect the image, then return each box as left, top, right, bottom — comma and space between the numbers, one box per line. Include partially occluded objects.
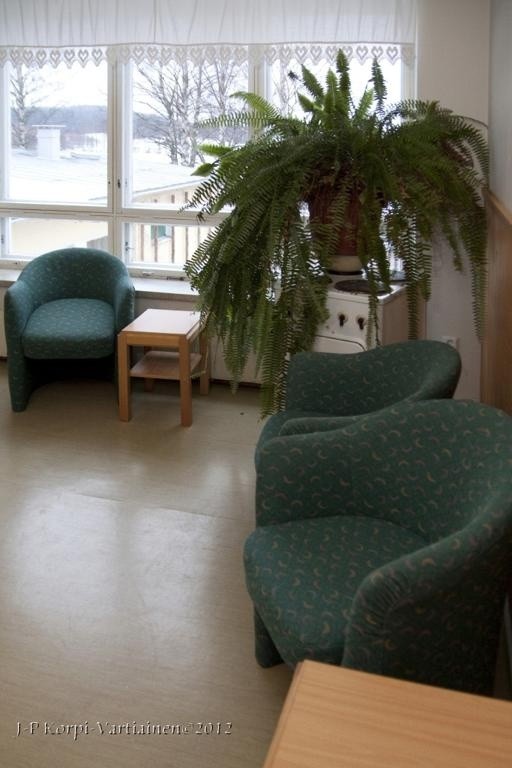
244, 399, 512, 676
254, 339, 462, 469
3, 247, 134, 412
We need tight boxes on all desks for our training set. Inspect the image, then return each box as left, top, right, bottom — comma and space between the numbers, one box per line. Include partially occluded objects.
263, 658, 512, 768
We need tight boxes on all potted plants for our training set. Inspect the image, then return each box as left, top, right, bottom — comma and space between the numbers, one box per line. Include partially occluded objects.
177, 48, 490, 420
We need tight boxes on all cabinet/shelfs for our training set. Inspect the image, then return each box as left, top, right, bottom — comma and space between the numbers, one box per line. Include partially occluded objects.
285, 273, 423, 354
